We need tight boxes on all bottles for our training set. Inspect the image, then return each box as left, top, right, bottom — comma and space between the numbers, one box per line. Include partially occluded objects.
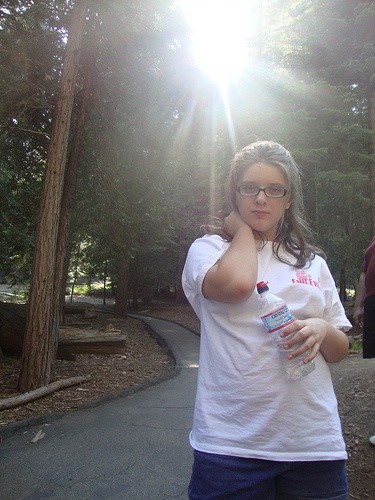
257, 281, 315, 380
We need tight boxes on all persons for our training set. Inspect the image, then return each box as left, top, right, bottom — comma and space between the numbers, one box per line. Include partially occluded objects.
182, 141, 352, 499
352, 235, 375, 359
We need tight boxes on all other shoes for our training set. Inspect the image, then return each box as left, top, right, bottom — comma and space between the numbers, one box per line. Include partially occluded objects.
368, 435, 375, 444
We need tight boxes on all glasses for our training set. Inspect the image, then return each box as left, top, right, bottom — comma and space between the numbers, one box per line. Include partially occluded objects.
236, 183, 288, 199
